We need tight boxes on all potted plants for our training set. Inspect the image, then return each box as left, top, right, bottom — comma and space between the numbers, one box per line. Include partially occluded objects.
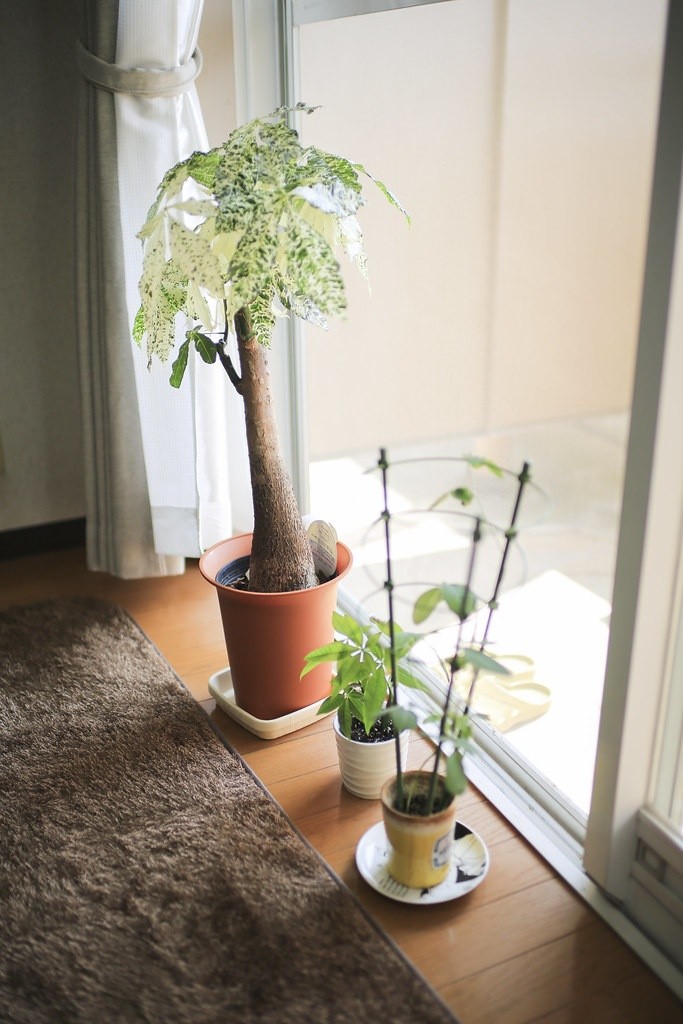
355, 441, 558, 905
130, 97, 410, 738
301, 608, 439, 801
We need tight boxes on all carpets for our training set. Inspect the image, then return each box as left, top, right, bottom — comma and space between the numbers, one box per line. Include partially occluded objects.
0, 595, 462, 1024
